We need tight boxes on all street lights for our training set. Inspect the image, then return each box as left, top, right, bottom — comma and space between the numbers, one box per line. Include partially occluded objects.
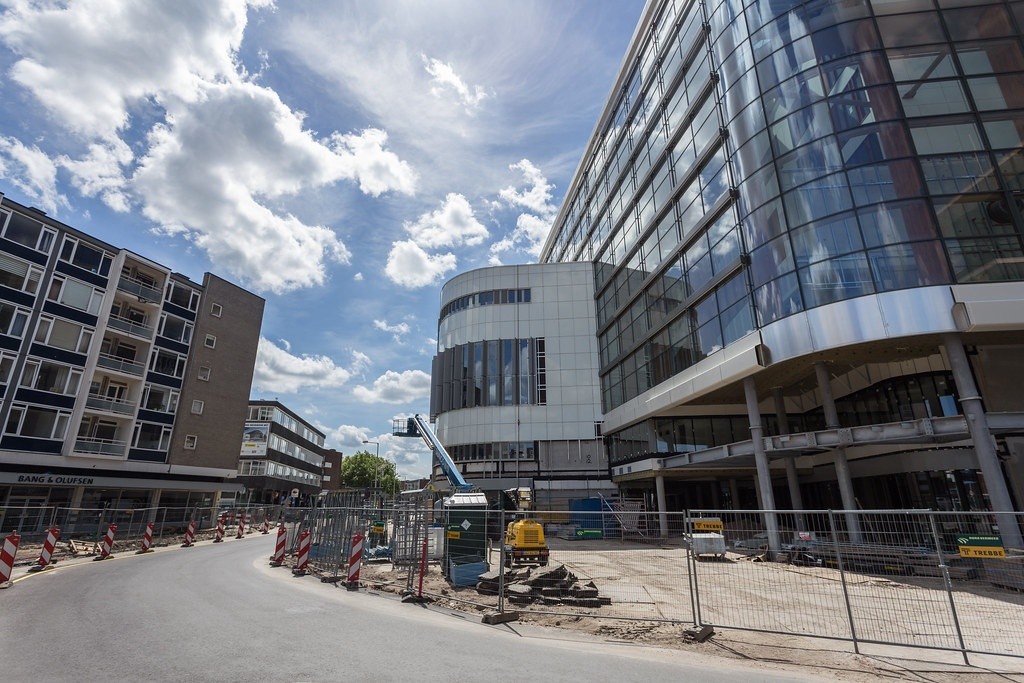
363, 440, 379, 507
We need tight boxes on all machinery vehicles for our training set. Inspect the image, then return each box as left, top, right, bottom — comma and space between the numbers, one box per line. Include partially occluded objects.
503, 519, 550, 567
